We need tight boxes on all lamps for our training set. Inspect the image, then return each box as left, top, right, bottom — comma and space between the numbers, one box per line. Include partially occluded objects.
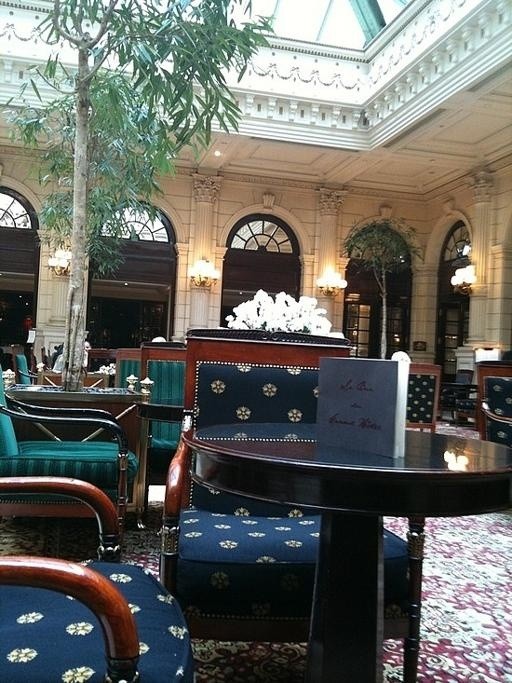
190, 261, 219, 289
47, 249, 71, 275
451, 264, 477, 295
315, 271, 347, 297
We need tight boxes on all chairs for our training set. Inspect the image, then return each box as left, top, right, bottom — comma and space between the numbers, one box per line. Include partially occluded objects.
0, 329, 512, 535
2, 476, 193, 683
160, 336, 425, 679
